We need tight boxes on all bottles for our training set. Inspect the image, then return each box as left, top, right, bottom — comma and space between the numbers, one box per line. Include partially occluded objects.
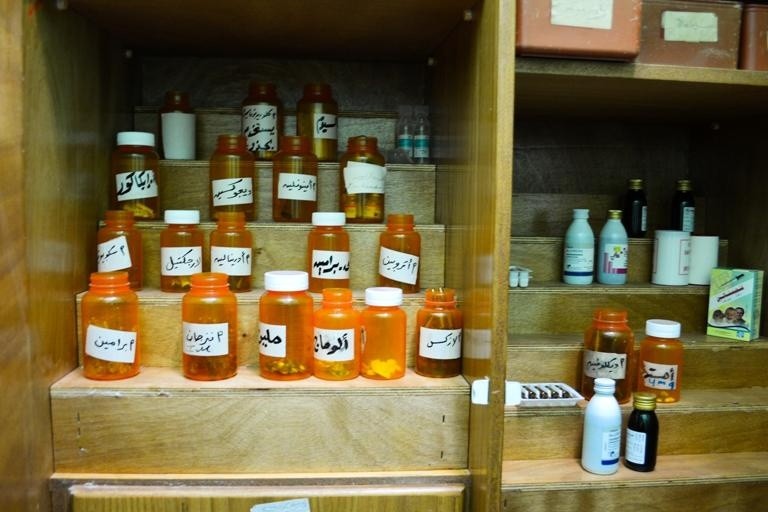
340, 135, 386, 223
361, 287, 406, 380
259, 271, 314, 381
417, 289, 462, 378
211, 212, 252, 292
638, 319, 683, 402
112, 133, 161, 220
583, 309, 633, 404
297, 84, 338, 160
159, 92, 196, 159
81, 271, 139, 381
210, 135, 254, 222
626, 179, 649, 237
309, 212, 349, 292
626, 392, 660, 473
381, 214, 420, 294
598, 210, 629, 286
414, 104, 430, 163
582, 378, 622, 475
98, 210, 141, 292
160, 210, 205, 293
273, 136, 318, 222
395, 105, 414, 163
242, 83, 284, 159
564, 208, 594, 285
183, 275, 237, 382
314, 289, 361, 381
674, 180, 694, 236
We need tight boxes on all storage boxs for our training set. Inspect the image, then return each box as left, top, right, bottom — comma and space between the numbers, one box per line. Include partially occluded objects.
515, 0, 643, 63
741, 4, 768, 71
637, 0, 744, 70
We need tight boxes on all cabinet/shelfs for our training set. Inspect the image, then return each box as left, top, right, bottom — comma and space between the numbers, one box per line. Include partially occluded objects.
490, 72, 768, 493
13, 1, 508, 473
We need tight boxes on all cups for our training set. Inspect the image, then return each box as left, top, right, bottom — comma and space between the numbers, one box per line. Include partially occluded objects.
650, 229, 691, 288
689, 234, 721, 287
